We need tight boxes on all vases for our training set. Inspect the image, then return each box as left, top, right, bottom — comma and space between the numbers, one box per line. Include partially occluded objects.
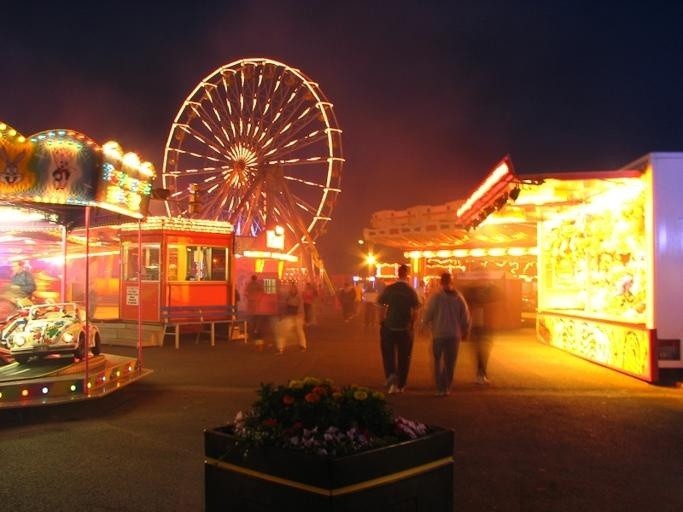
203, 415, 458, 511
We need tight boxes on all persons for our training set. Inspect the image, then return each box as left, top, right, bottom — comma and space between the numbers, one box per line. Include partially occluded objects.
415, 281, 425, 303
423, 278, 435, 303
274, 280, 307, 356
301, 282, 314, 327
246, 275, 264, 339
333, 280, 378, 327
421, 272, 471, 398
378, 262, 423, 395
462, 267, 503, 387
9, 260, 34, 299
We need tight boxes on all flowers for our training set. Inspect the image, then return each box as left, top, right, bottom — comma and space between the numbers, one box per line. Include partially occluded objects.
233, 376, 429, 456
